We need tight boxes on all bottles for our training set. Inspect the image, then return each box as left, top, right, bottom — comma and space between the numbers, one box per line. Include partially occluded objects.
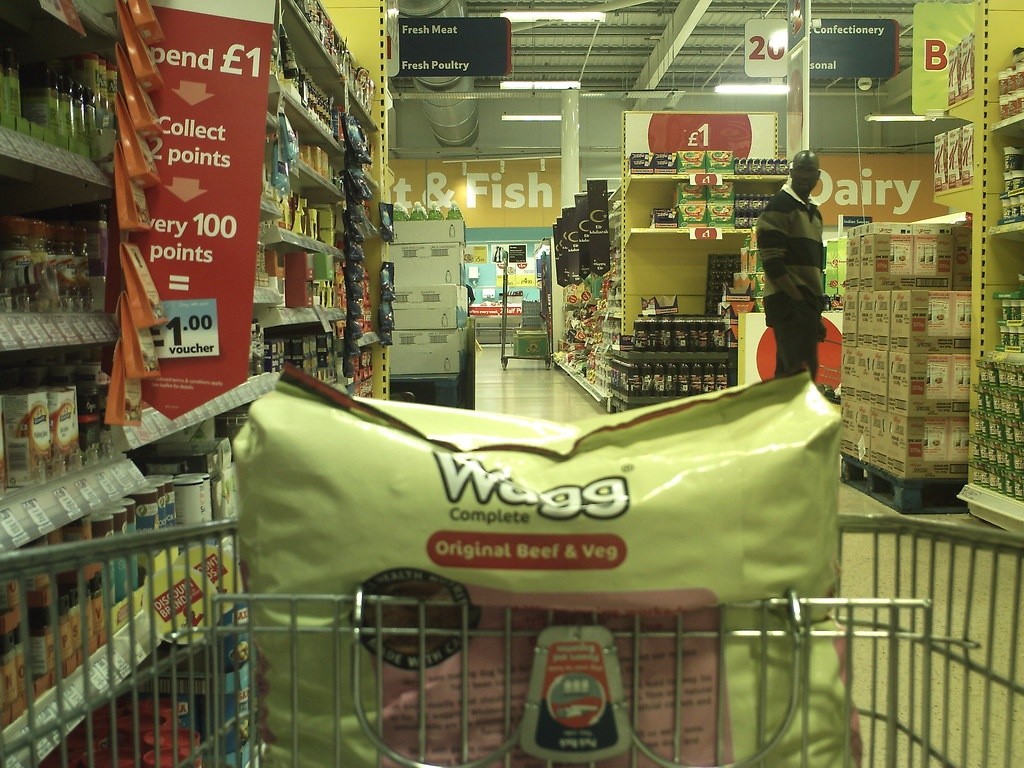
393, 200, 462, 220
0, 45, 95, 139
1, 216, 93, 313
299, 0, 343, 129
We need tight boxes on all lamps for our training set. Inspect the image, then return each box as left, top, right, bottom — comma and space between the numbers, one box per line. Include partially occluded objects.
500, 161, 505, 174
500, 80, 581, 90
864, 114, 927, 122
540, 159, 546, 171
499, 10, 606, 23
462, 163, 467, 176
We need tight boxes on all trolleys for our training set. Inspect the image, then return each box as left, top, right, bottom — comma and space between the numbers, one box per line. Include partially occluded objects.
0, 506, 1024, 768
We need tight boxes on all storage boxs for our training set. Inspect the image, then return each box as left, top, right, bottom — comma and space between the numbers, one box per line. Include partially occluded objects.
391, 284, 468, 330
605, 31, 974, 479
389, 219, 466, 244
390, 327, 468, 375
517, 330, 548, 357
388, 242, 464, 287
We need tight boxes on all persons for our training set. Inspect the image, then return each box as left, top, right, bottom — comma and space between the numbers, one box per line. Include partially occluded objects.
755, 150, 827, 381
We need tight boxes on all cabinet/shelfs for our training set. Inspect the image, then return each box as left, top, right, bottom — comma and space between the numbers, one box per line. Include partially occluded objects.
933, 0, 1024, 532
0, 0, 390, 768
552, 110, 791, 414
